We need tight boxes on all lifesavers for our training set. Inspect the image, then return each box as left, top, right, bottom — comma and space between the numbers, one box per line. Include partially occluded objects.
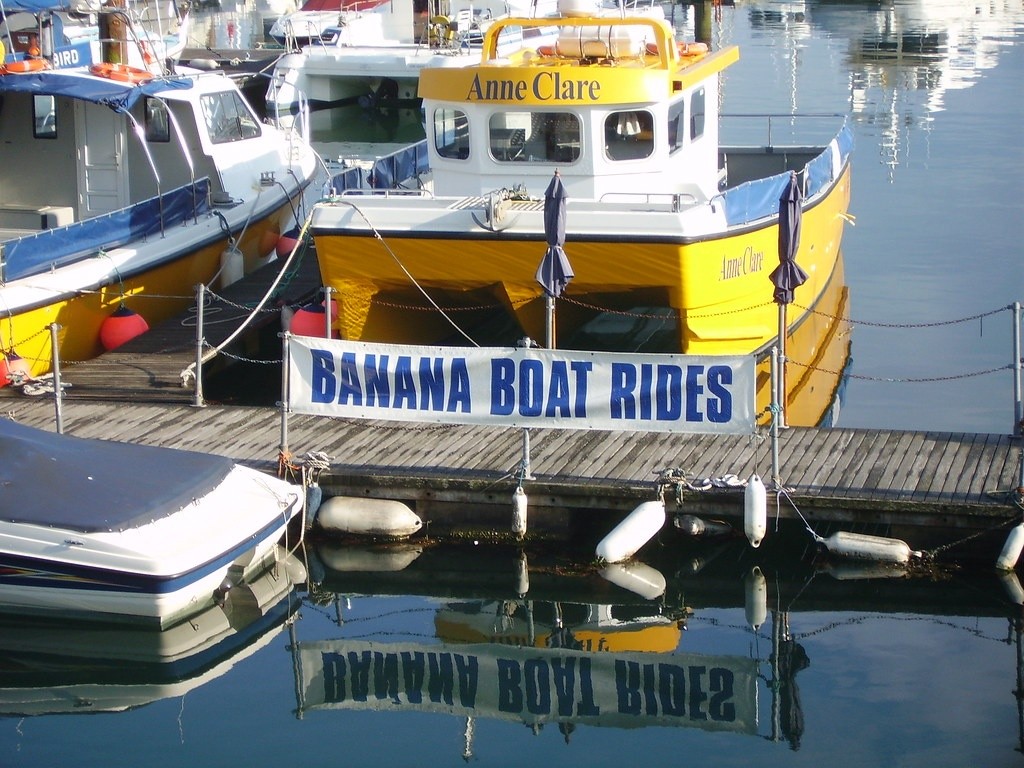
427, 14, 454, 45
1, 59, 43, 75
89, 64, 153, 83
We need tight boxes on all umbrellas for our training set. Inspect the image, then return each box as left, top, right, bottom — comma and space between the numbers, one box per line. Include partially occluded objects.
769, 171, 809, 429
534, 170, 576, 350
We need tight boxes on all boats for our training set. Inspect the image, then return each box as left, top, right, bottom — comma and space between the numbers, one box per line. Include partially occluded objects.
0, 0, 858, 633
